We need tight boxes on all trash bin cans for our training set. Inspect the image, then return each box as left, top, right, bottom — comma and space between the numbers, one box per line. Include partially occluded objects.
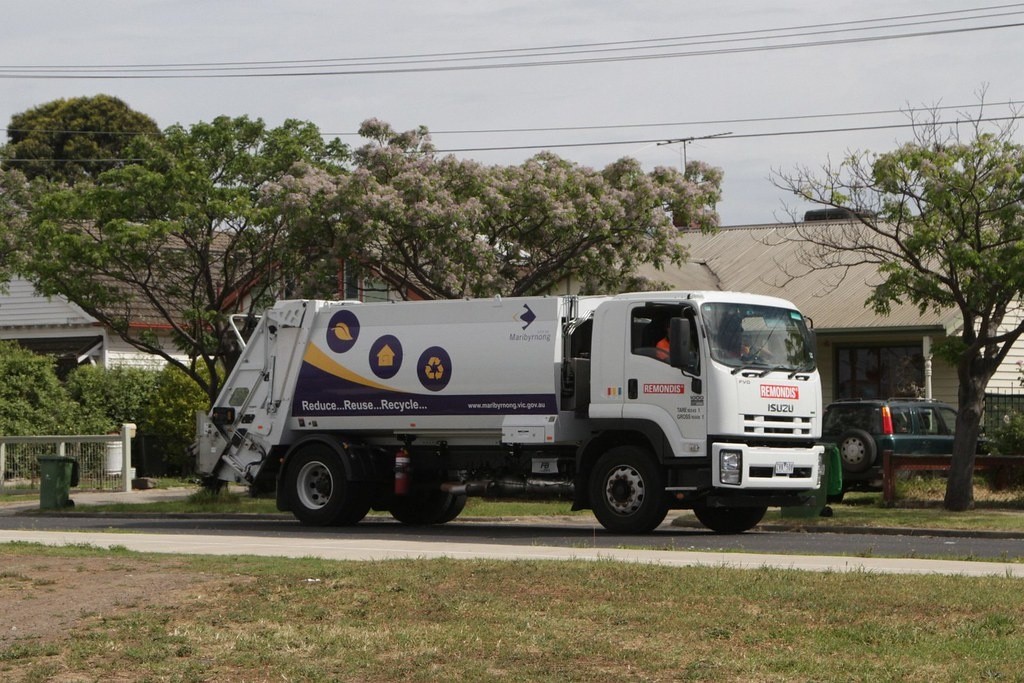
779, 441, 844, 520
35, 455, 80, 511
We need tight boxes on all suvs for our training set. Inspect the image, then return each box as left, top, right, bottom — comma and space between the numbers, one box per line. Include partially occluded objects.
821, 396, 995, 494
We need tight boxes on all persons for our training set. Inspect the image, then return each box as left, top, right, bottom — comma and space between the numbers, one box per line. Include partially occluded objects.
657, 324, 670, 363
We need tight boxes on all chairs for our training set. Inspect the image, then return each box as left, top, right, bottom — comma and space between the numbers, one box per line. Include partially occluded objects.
918, 415, 930, 430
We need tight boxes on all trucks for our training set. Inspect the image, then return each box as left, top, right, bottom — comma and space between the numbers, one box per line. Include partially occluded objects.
191, 289, 827, 537
